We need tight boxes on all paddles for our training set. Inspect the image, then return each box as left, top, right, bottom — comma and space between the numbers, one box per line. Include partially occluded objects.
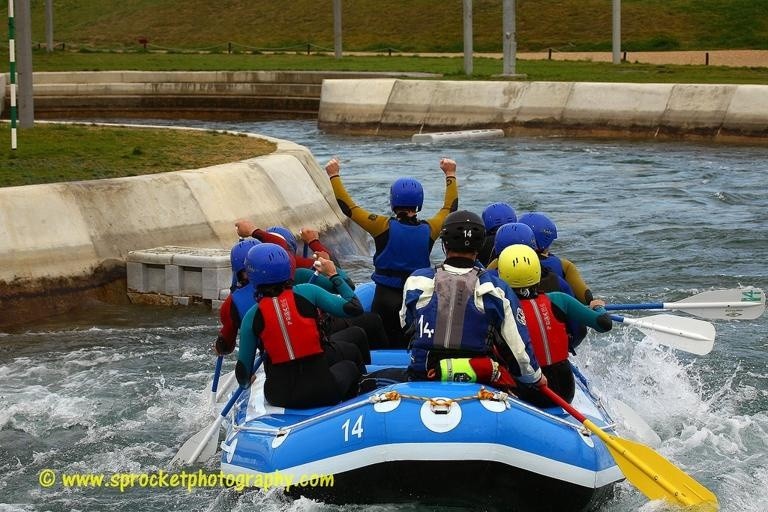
601, 286, 768, 322
538, 383, 719, 512
166, 356, 264, 470
607, 310, 718, 356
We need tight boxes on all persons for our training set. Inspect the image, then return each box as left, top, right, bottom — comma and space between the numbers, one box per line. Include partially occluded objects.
267, 226, 389, 350
323, 157, 460, 350
486, 222, 593, 349
291, 251, 372, 366
213, 220, 368, 375
234, 243, 363, 410
518, 212, 594, 307
358, 209, 548, 399
491, 243, 613, 409
476, 202, 518, 269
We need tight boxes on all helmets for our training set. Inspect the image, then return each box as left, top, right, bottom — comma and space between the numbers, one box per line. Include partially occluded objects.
495, 223, 539, 256
442, 211, 486, 254
389, 178, 423, 209
230, 238, 263, 273
243, 244, 291, 289
497, 246, 542, 288
517, 214, 557, 252
482, 204, 517, 233
265, 228, 298, 254
